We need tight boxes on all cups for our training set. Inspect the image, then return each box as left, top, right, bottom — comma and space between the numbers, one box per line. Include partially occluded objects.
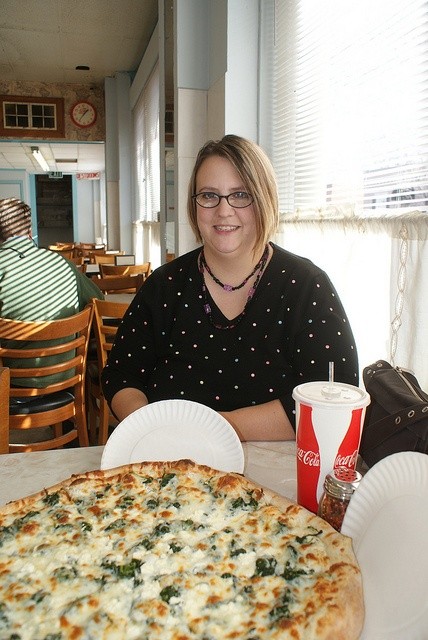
290, 378, 372, 522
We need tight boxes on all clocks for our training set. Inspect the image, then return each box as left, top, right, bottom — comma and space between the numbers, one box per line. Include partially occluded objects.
70, 100, 97, 129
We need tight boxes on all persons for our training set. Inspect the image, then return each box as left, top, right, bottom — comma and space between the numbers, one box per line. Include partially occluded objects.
0, 197, 106, 405
100, 134, 360, 446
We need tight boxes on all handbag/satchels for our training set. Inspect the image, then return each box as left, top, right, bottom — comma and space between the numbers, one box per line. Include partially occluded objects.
358, 359, 427, 467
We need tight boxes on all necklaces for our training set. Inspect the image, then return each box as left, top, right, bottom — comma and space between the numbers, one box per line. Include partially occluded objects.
198, 244, 269, 331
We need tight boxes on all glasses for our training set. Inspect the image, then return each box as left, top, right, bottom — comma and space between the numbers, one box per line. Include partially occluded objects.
190, 192, 255, 209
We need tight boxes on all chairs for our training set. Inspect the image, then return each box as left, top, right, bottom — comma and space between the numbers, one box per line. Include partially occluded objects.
93, 274, 144, 294
0, 301, 96, 456
98, 262, 152, 276
86, 296, 142, 446
46, 241, 127, 264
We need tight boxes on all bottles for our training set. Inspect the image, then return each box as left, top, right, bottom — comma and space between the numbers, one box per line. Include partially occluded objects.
317, 469, 358, 530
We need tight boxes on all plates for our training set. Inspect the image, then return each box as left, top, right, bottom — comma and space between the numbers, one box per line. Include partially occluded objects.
101, 397, 244, 479
338, 451, 428, 638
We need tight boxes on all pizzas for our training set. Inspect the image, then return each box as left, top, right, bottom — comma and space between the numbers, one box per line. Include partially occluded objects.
0, 457, 365, 640
